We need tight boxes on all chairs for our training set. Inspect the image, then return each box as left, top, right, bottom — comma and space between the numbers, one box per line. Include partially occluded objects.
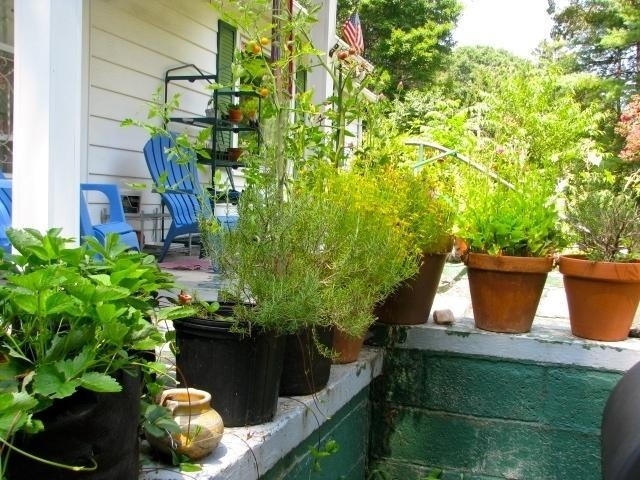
1, 167, 147, 265
143, 128, 233, 273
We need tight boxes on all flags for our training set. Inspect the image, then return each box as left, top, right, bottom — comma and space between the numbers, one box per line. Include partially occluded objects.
341, 7, 366, 56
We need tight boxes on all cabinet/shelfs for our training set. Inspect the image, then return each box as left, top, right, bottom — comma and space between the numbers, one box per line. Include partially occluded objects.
164, 61, 264, 254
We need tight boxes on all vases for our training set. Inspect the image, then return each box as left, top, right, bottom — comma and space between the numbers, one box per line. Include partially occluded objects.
144, 386, 227, 466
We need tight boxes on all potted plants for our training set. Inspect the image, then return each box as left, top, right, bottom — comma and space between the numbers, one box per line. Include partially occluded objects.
214, 104, 252, 163
559, 170, 640, 344
171, 187, 289, 427
1, 220, 167, 480
459, 166, 555, 334
207, 126, 448, 397
